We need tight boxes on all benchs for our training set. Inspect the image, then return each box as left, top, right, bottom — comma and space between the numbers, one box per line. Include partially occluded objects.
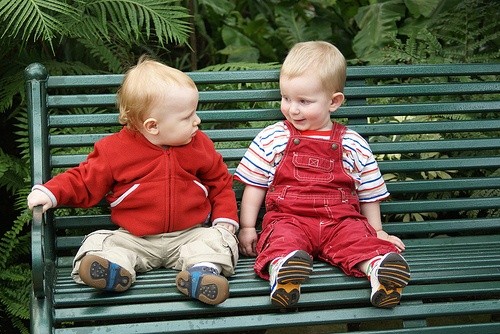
23, 63, 500, 334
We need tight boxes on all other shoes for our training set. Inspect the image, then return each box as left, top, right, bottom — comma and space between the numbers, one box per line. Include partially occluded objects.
79, 255, 132, 292
270, 250, 313, 308
175, 266, 230, 306
370, 252, 411, 309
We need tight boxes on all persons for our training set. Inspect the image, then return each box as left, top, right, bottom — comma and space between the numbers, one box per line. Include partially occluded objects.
27, 54, 239, 305
234, 41, 411, 308
